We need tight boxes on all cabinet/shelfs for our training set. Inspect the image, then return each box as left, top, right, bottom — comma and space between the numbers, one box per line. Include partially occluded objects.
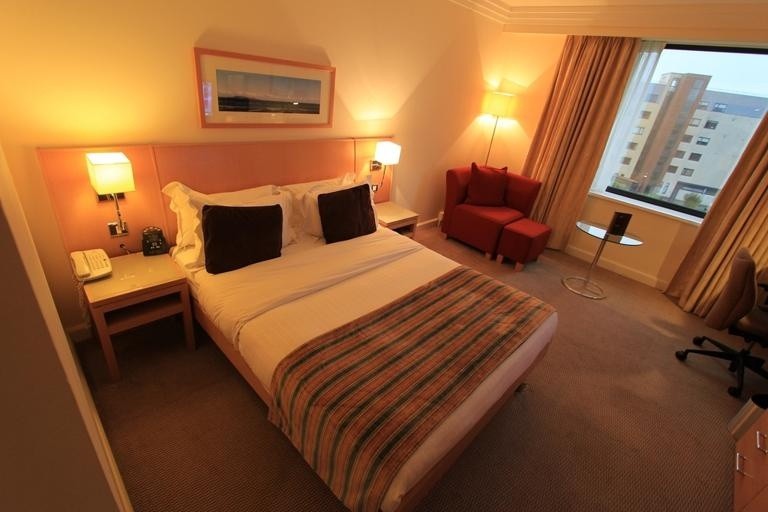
733, 409, 768, 512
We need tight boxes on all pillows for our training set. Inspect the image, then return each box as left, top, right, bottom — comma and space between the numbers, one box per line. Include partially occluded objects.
185, 190, 293, 273
318, 184, 376, 244
465, 162, 508, 206
302, 176, 379, 240
280, 172, 356, 236
162, 181, 280, 257
200, 204, 283, 274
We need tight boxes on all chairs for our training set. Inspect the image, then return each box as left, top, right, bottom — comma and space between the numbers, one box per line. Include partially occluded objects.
441, 165, 542, 260
675, 248, 768, 399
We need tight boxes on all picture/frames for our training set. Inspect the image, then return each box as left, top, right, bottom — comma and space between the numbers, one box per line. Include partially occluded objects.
193, 47, 336, 128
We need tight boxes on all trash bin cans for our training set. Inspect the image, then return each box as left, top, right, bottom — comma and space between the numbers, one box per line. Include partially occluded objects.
727, 393, 768, 441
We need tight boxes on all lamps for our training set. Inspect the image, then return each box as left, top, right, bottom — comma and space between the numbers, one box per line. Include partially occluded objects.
85, 151, 134, 236
483, 91, 516, 165
370, 141, 401, 192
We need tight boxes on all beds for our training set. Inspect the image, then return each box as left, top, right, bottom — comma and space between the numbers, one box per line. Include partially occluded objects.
171, 227, 558, 512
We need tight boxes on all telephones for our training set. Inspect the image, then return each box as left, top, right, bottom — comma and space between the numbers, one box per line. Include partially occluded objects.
70, 248, 112, 282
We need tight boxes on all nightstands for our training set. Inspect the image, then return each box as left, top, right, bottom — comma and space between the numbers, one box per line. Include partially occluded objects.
375, 201, 418, 239
80, 251, 195, 383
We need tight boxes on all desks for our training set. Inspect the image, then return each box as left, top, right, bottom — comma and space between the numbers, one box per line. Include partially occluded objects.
562, 219, 644, 300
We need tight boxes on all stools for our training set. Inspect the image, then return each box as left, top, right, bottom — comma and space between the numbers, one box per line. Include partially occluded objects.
495, 217, 552, 271
